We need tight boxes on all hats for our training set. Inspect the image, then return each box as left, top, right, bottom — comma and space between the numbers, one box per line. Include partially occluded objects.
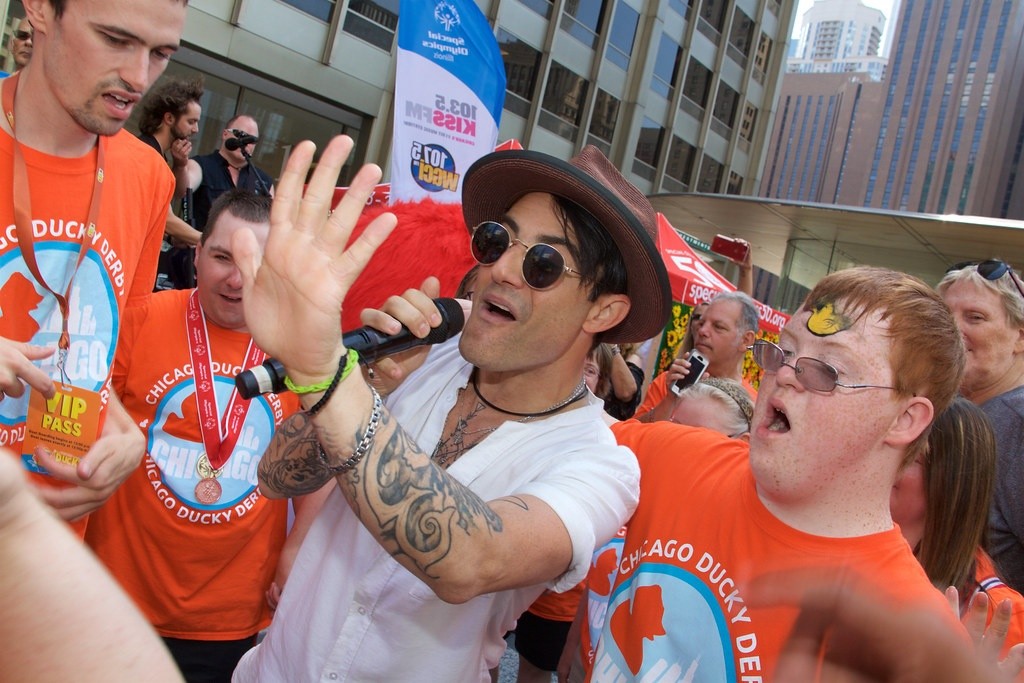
462, 144, 672, 344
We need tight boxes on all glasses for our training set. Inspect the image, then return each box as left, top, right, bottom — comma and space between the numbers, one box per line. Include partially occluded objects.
227, 129, 259, 144
470, 221, 603, 290
946, 258, 1024, 296
691, 314, 701, 320
746, 338, 909, 392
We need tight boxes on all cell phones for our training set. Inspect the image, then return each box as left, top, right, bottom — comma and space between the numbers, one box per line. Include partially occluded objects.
710, 235, 749, 264
670, 349, 711, 397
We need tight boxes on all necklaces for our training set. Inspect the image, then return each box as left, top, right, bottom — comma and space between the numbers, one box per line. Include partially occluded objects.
431, 376, 587, 466
471, 367, 588, 422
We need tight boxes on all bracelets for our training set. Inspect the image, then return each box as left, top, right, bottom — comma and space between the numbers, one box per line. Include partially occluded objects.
299, 350, 374, 416
317, 382, 381, 473
282, 347, 358, 394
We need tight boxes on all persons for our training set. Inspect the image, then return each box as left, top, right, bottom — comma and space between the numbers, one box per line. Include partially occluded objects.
0, 0, 1024, 683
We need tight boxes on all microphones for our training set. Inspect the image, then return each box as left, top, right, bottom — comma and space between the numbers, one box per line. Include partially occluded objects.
225, 135, 257, 151
234, 297, 465, 399
